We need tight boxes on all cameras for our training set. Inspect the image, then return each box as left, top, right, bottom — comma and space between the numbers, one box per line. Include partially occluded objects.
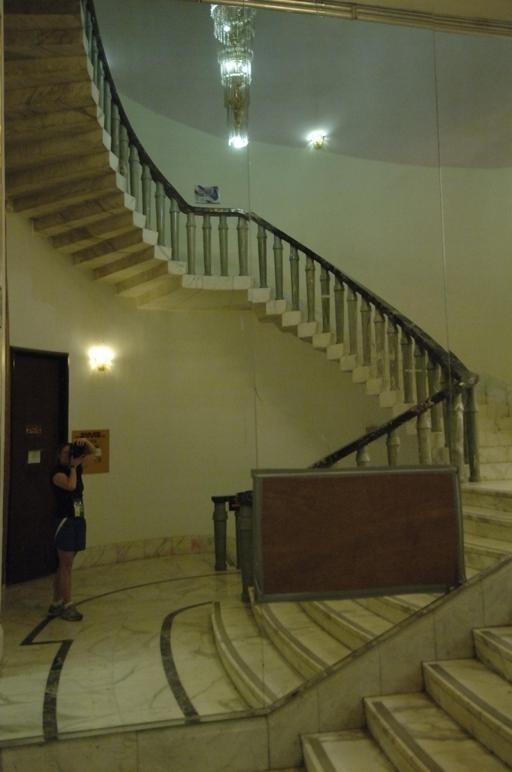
69, 442, 89, 458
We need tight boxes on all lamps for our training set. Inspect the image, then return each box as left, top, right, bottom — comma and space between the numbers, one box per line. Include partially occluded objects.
209, 3, 258, 151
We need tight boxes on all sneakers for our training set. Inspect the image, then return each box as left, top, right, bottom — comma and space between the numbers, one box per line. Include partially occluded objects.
47, 603, 83, 621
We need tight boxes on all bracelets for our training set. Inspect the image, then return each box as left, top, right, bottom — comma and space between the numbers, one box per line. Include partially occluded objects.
69, 464, 77, 469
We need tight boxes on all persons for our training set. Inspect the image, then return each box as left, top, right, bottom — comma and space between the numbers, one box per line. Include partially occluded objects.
50, 436, 94, 620
